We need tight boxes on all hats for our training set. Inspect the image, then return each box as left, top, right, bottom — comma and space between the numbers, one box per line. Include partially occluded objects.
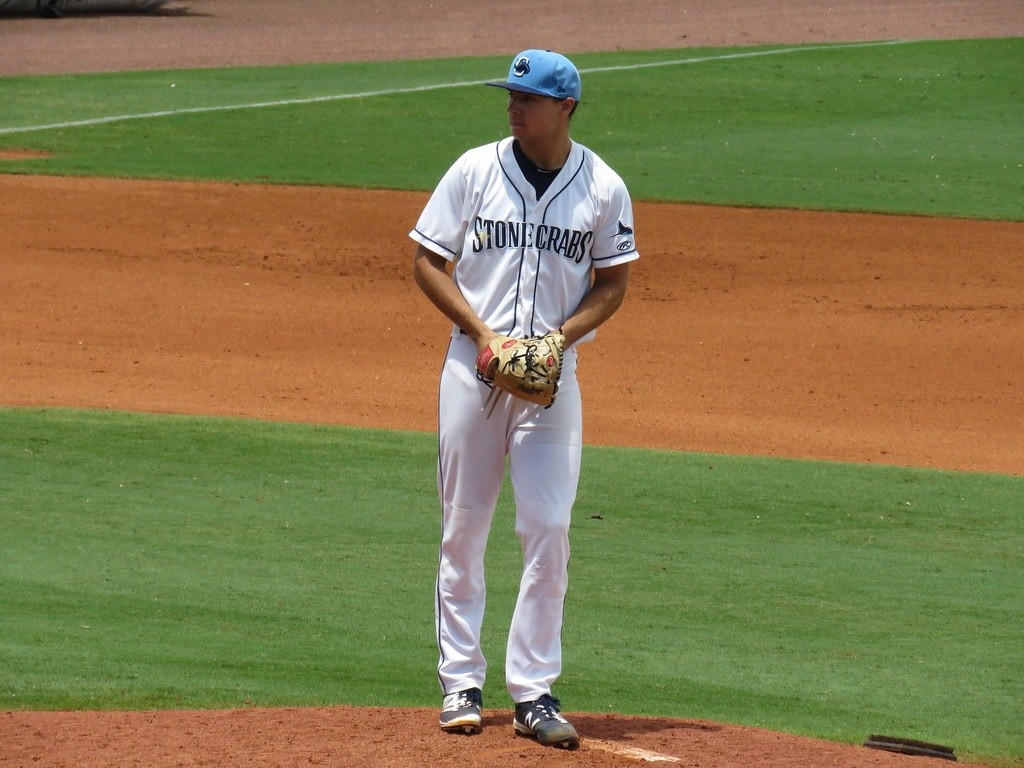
485, 49, 581, 101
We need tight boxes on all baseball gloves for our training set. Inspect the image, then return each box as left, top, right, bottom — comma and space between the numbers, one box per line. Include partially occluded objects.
475, 330, 567, 423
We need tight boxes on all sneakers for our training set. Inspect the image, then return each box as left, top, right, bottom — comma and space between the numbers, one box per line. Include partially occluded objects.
512, 694, 579, 750
439, 687, 482, 735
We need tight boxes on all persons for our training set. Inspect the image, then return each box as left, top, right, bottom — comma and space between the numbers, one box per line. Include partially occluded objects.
408, 47, 639, 749
